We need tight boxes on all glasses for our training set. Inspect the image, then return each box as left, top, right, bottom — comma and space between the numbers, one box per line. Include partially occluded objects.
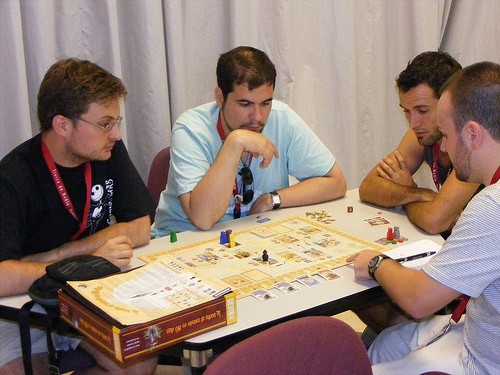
241, 167, 254, 204
75, 115, 123, 132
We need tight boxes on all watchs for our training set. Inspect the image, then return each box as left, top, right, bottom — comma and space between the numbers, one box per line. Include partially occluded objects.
269, 190, 281, 210
368, 254, 392, 281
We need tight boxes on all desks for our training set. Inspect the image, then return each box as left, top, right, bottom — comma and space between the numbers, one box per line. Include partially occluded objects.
0, 187, 446, 375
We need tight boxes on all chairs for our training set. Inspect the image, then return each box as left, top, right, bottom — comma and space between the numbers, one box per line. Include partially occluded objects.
148, 147, 170, 195
201, 316, 373, 375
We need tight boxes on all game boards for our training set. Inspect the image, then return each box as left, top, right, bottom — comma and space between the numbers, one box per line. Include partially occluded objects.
136, 215, 393, 301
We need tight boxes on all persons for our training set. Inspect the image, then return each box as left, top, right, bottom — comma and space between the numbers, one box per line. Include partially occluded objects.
151, 46, 347, 241
348, 51, 486, 336
0, 57, 159, 375
347, 61, 500, 374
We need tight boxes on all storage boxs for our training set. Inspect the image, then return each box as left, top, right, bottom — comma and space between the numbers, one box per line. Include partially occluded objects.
57, 264, 238, 369
